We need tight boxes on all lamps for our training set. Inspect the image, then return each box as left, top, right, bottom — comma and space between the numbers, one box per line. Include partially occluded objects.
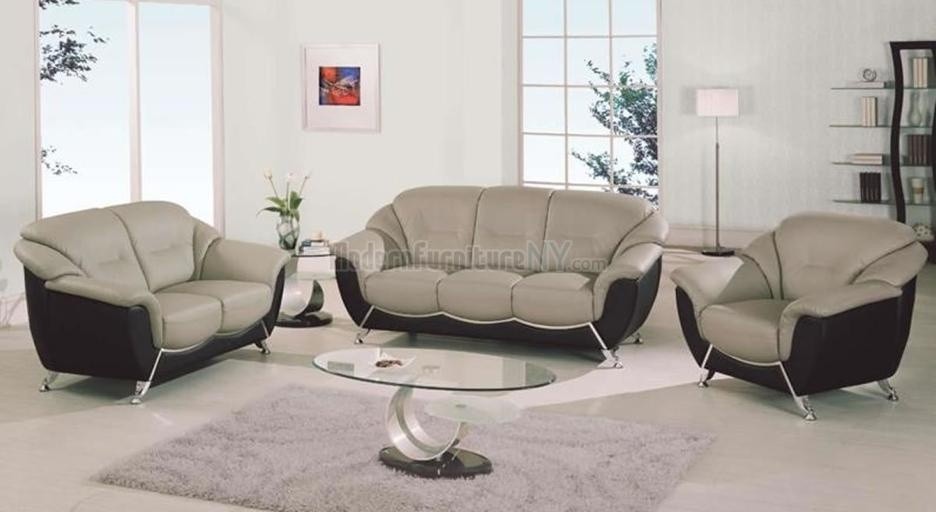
695, 88, 739, 256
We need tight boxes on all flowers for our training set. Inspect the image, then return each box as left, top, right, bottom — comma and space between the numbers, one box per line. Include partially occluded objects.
255, 170, 314, 222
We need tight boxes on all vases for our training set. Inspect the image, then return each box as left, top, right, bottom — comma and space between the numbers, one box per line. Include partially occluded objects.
273, 213, 299, 249
908, 93, 922, 127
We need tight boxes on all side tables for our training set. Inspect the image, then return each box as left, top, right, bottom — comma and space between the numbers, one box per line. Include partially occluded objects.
275, 246, 334, 327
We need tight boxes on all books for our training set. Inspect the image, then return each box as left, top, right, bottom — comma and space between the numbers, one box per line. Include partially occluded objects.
850, 57, 936, 204
298, 238, 333, 256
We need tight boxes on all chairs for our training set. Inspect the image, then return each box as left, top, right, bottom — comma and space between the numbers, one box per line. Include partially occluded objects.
670, 210, 929, 421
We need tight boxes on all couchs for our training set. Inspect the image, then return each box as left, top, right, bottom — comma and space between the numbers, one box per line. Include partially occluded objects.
330, 185, 669, 369
14, 202, 291, 404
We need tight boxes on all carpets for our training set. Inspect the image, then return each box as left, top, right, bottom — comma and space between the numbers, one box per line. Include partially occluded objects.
88, 380, 714, 512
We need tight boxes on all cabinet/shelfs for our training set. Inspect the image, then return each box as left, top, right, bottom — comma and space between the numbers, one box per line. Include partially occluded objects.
829, 41, 936, 263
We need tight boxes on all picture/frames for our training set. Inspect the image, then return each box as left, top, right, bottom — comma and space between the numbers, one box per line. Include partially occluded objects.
300, 43, 381, 133
